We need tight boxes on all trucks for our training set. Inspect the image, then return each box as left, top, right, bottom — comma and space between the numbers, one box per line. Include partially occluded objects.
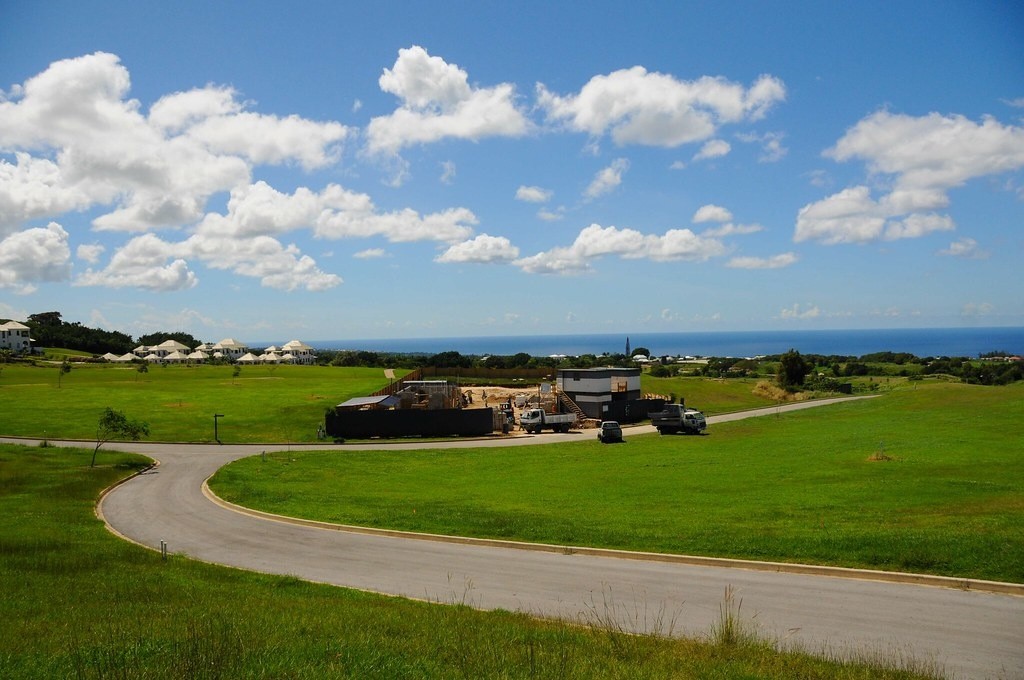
648, 403, 684, 420
651, 410, 707, 435
600, 420, 623, 443
519, 406, 578, 434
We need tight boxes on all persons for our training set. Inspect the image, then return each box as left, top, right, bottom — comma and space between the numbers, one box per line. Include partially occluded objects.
455, 388, 533, 435
